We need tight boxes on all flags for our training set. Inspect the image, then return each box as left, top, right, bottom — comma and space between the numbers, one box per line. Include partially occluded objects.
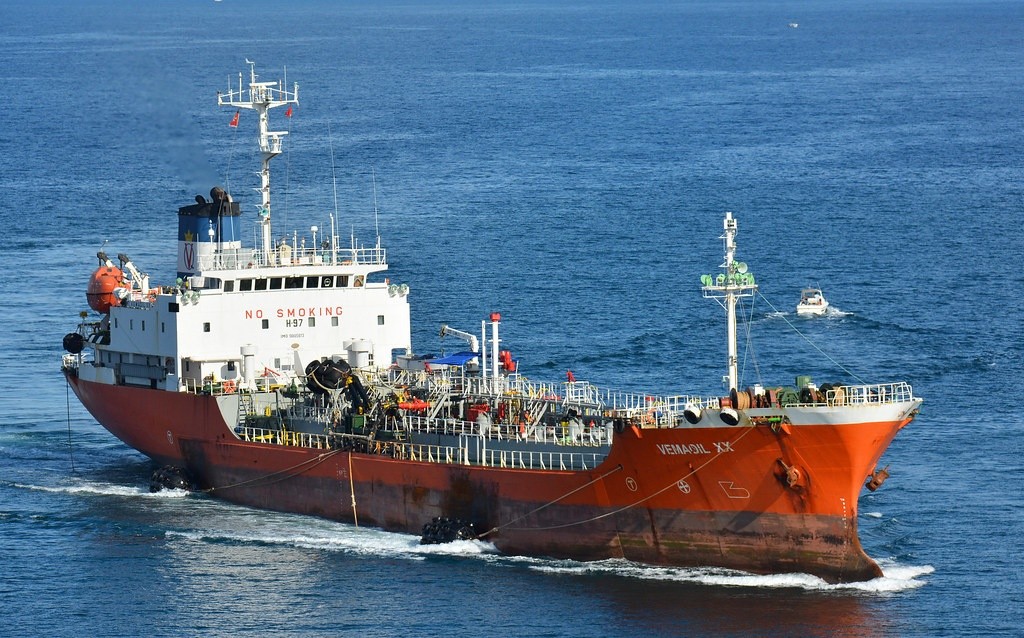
284, 107, 292, 118
229, 111, 239, 127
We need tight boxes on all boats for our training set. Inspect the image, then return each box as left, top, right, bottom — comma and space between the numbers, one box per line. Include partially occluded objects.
793, 285, 828, 319
61, 52, 923, 587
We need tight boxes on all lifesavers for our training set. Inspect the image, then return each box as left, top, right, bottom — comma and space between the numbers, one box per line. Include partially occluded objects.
614, 418, 625, 433
224, 381, 236, 393
147, 290, 158, 302
